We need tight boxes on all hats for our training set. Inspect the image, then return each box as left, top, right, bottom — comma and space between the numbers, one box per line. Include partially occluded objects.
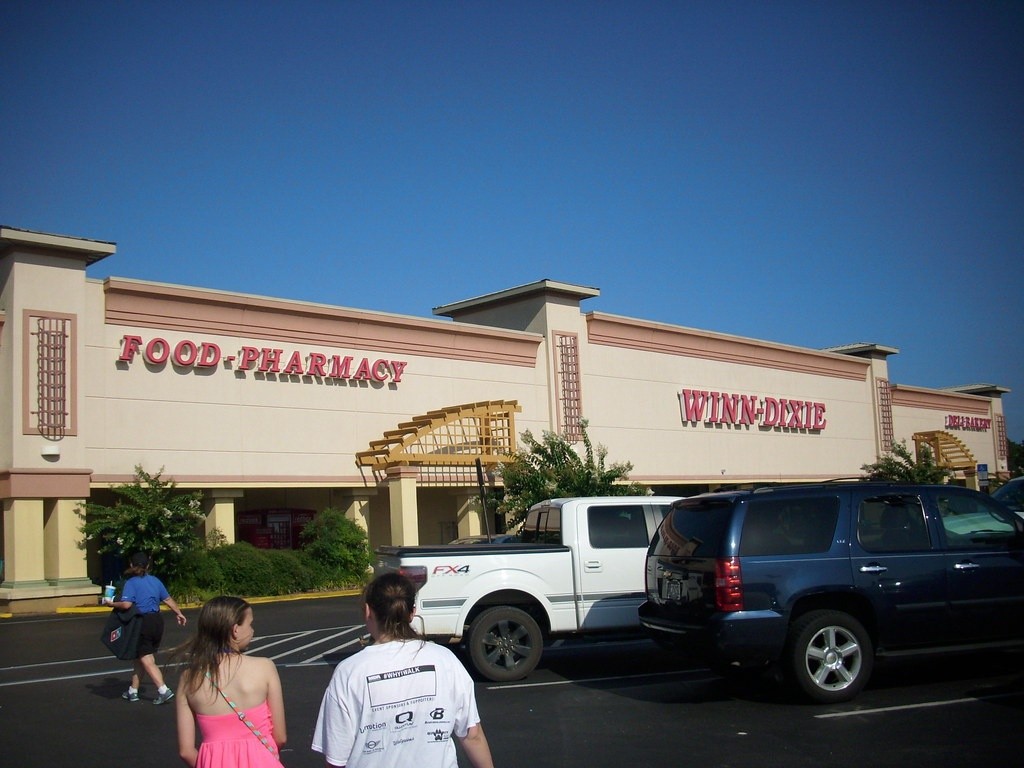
129, 551, 148, 567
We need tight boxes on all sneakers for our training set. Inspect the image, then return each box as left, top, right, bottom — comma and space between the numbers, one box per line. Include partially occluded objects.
152, 688, 175, 704
122, 690, 139, 702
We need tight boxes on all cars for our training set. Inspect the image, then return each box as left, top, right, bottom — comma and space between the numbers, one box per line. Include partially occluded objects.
447, 534, 522, 544
634, 475, 1024, 702
940, 475, 1024, 537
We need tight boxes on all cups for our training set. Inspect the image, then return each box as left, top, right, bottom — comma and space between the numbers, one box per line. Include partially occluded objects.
105, 586, 116, 597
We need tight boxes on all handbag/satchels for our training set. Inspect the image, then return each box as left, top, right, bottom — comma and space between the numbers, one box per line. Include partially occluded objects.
100, 600, 144, 660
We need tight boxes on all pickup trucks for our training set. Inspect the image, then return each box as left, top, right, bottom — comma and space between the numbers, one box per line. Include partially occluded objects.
372, 495, 691, 683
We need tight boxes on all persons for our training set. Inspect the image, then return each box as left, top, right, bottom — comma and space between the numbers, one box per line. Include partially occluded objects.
103, 551, 186, 704
176, 595, 287, 768
311, 573, 494, 768
